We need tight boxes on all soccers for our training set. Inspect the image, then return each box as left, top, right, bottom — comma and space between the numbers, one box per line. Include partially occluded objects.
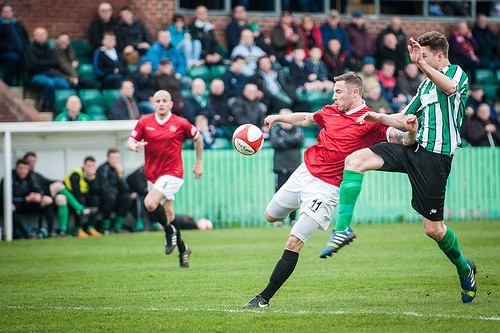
197, 218, 213, 231
232, 123, 265, 156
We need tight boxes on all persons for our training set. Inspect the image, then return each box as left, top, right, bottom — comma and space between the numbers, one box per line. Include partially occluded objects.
0, 158, 56, 239
244, 70, 418, 310
126, 89, 204, 268
320, 30, 478, 304
269, 108, 306, 227
52, 95, 90, 121
23, 27, 71, 112
53, 33, 80, 90
87, 2, 499, 148
95, 149, 137, 234
64, 153, 105, 237
0, 3, 31, 86
22, 152, 100, 237
126, 166, 160, 231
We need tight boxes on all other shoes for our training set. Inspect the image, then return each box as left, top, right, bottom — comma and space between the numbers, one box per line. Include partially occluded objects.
25, 228, 130, 239
80, 208, 98, 217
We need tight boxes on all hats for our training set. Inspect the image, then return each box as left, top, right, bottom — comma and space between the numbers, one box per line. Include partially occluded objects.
361, 57, 376, 66
138, 57, 152, 67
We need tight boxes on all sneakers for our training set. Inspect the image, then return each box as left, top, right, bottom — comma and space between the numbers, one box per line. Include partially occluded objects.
459, 260, 477, 304
242, 295, 269, 309
319, 227, 357, 259
179, 245, 191, 269
165, 225, 178, 254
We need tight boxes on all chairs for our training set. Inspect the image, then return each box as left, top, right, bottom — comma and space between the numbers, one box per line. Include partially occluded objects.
260, 90, 328, 148
476, 69, 500, 103
32, 37, 234, 149
